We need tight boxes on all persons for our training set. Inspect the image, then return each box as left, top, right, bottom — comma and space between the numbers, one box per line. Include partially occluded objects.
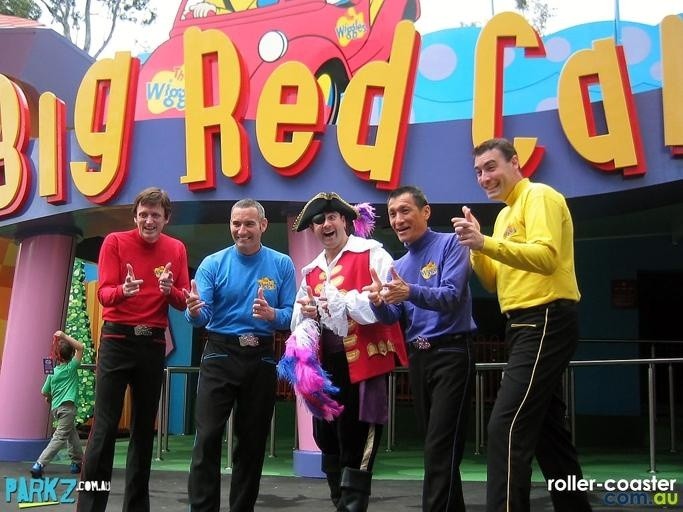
180, 195, 298, 512
448, 138, 594, 512
76, 186, 192, 512
29, 328, 84, 478
285, 188, 410, 511
357, 182, 478, 512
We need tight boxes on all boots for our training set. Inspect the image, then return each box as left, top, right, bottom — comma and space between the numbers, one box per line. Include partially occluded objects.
321, 455, 371, 511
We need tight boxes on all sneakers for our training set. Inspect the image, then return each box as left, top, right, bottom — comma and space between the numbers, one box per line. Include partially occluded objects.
30, 462, 42, 478
70, 464, 80, 473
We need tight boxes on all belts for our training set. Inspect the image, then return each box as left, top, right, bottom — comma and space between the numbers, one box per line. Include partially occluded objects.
207, 332, 275, 347
408, 333, 466, 352
106, 322, 164, 336
504, 301, 572, 319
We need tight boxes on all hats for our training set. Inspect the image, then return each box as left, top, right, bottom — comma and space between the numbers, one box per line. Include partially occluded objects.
292, 192, 359, 235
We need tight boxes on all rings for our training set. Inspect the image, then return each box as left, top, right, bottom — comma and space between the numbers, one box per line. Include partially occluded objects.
162, 281, 164, 286
255, 309, 258, 313
459, 234, 465, 240
